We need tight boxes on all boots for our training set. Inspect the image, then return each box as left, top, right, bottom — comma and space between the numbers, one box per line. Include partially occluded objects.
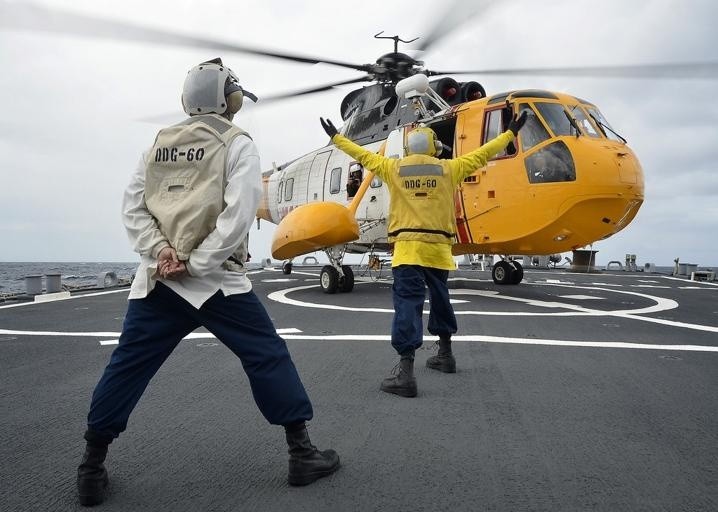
284, 421, 338, 485
78, 439, 107, 505
381, 350, 416, 397
427, 333, 456, 372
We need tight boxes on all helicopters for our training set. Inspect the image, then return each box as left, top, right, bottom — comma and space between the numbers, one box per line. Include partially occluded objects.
1, 3, 716, 293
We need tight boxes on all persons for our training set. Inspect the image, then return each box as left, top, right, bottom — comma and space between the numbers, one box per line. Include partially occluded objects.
319, 106, 529, 396
75, 52, 341, 506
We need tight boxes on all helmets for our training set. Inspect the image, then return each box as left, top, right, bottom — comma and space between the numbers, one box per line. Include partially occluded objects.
406, 127, 443, 156
182, 57, 244, 115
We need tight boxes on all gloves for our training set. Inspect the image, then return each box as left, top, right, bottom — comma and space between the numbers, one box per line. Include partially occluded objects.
508, 111, 527, 133
320, 118, 336, 136
